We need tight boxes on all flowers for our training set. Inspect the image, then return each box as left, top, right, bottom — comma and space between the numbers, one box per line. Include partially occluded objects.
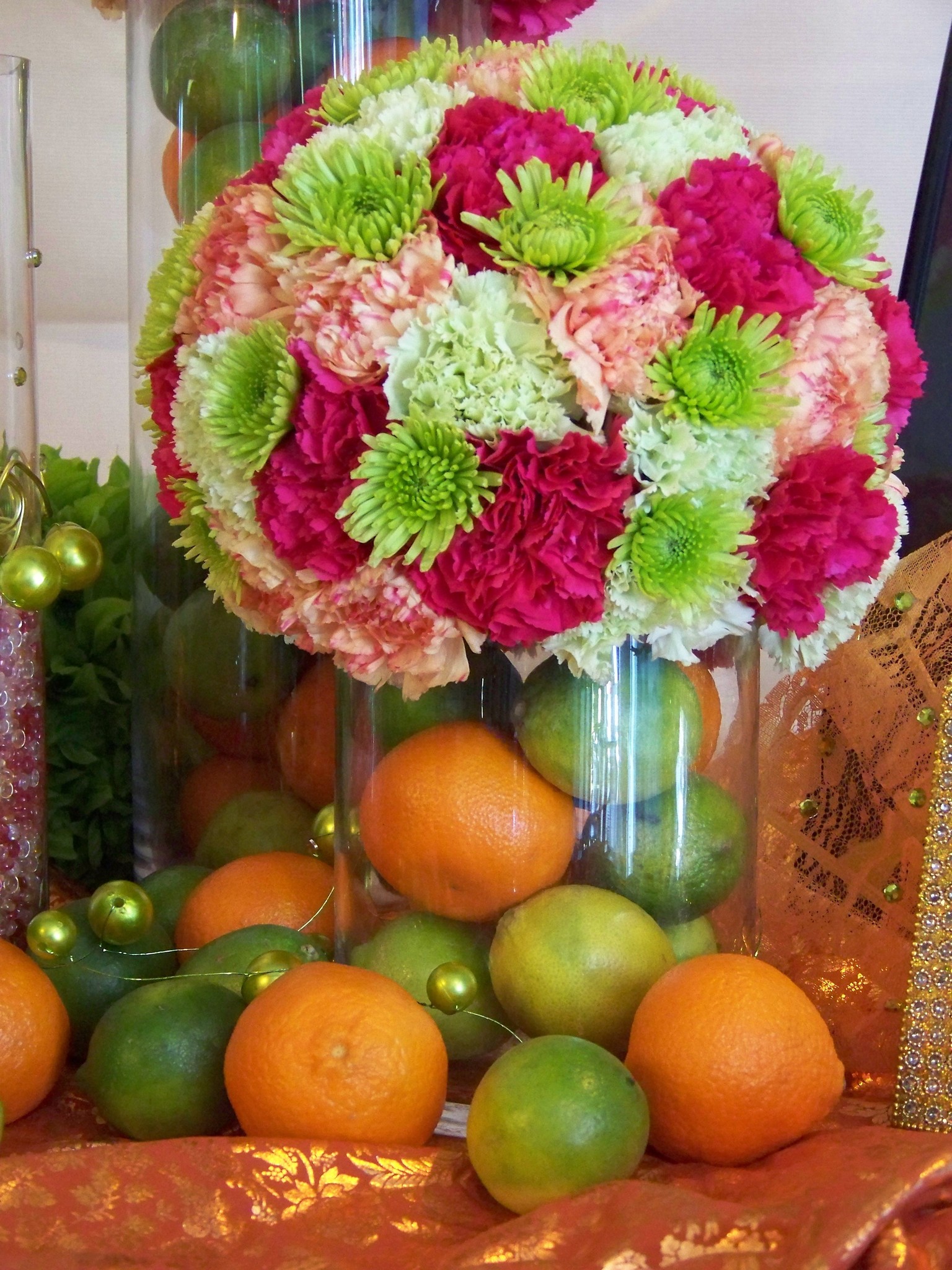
136, 34, 926, 705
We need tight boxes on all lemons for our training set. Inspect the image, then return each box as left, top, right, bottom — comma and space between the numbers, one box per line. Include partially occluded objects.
0, 508, 846, 1216
